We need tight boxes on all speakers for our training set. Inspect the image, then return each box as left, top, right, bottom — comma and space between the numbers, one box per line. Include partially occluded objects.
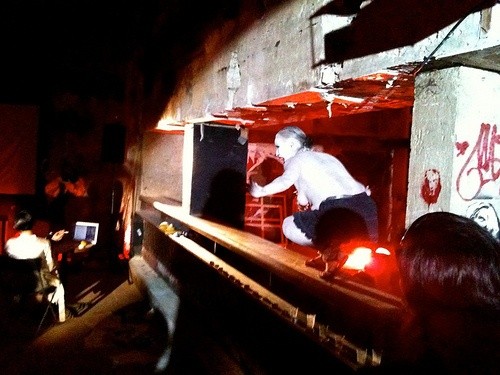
181, 123, 249, 229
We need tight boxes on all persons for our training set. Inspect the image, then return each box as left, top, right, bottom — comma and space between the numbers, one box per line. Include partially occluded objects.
4, 211, 59, 308
246, 126, 379, 279
396, 212, 500, 375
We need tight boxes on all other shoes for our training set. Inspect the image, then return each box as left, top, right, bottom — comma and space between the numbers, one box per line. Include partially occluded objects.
305, 255, 325, 270
319, 258, 345, 280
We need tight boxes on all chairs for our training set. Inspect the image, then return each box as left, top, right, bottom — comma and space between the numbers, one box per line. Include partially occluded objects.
6, 251, 57, 335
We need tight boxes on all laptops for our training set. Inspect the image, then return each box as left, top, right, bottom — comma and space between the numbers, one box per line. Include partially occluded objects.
72, 222, 100, 247
